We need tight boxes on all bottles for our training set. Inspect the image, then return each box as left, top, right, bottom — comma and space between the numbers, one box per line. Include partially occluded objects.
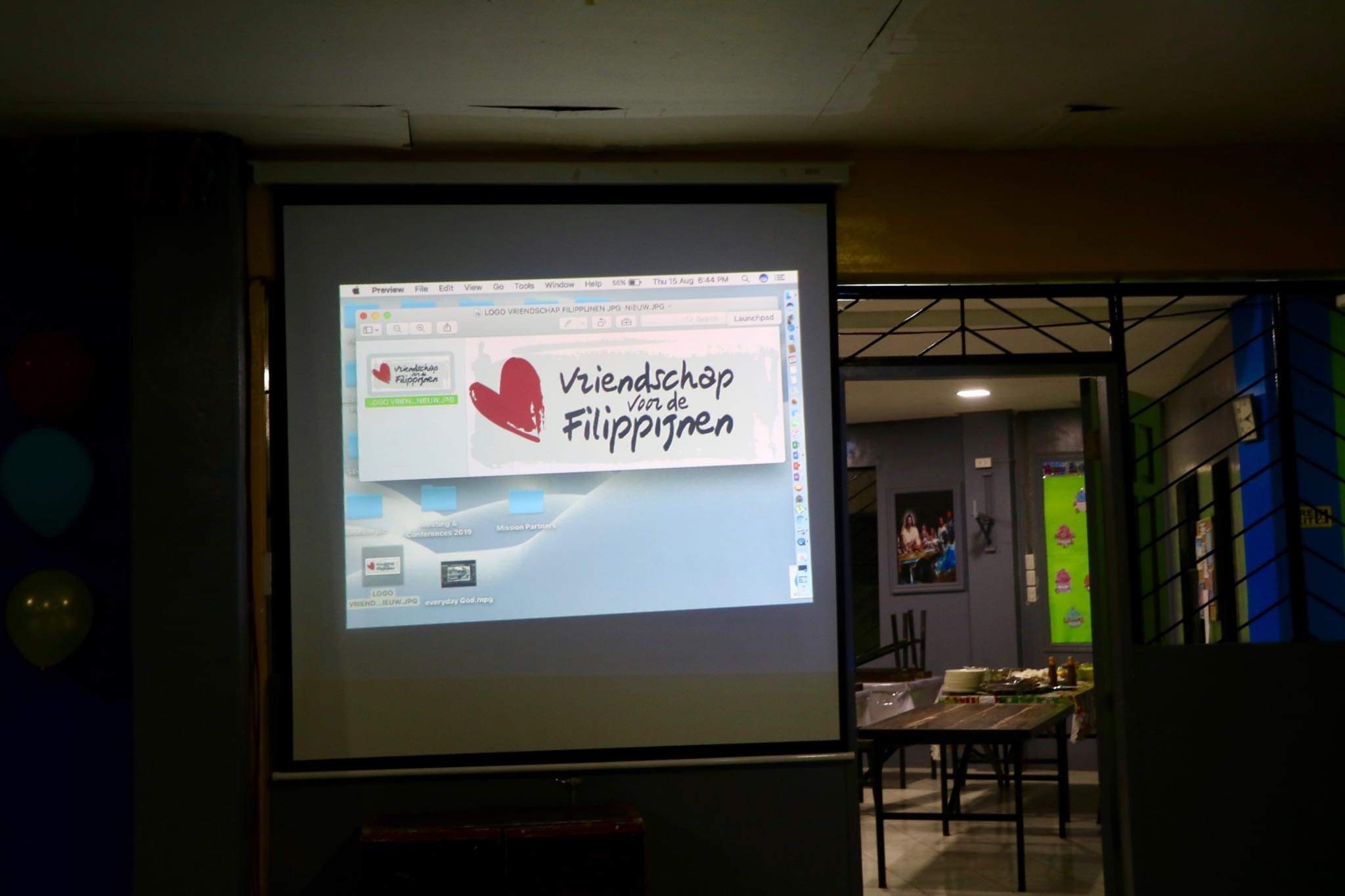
1048, 657, 1057, 686
1068, 656, 1077, 686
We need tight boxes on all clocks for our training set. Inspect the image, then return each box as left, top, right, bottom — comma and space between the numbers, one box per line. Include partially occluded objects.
1232, 393, 1260, 442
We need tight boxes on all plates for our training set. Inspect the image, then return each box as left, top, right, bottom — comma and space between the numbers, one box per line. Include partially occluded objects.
941, 670, 986, 694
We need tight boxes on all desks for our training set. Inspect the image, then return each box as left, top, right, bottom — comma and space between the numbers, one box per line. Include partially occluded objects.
898, 547, 937, 584
853, 700, 1076, 893
862, 675, 944, 764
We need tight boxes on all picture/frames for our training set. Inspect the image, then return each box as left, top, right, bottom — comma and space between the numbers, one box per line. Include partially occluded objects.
885, 482, 965, 594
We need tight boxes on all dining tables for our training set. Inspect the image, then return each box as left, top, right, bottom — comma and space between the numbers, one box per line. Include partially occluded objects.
938, 674, 1095, 837
